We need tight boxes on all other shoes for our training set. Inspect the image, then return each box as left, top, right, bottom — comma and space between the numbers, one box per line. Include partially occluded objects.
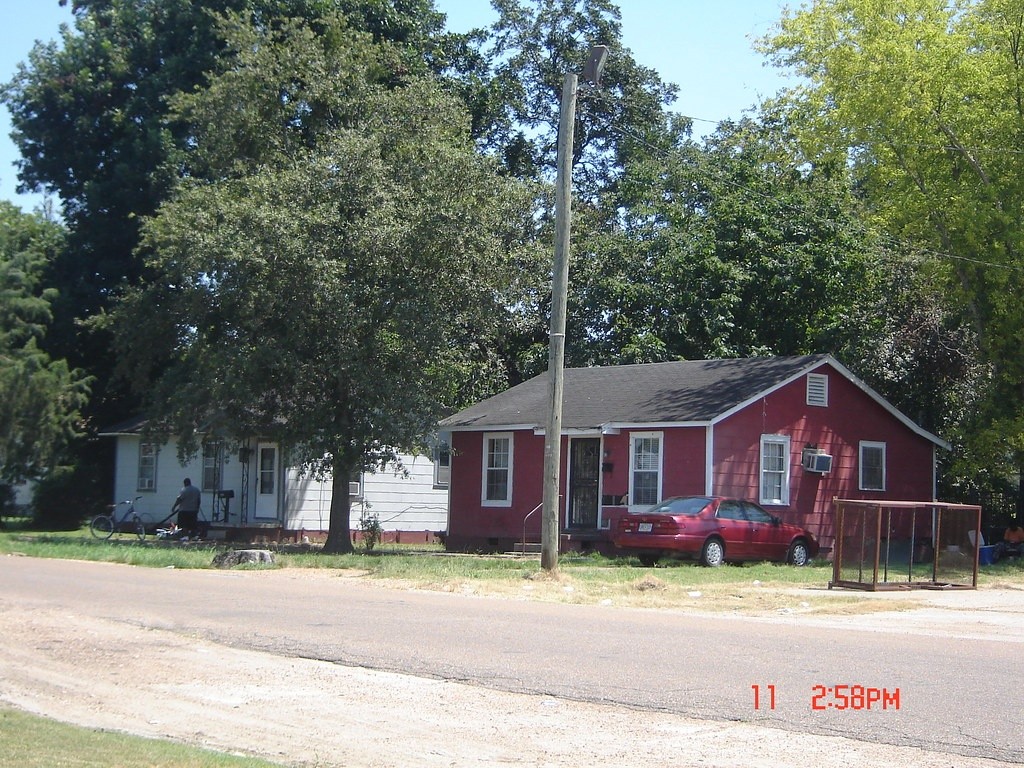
180, 536, 189, 541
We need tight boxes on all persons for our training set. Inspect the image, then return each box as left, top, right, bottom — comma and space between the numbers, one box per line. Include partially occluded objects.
171, 477, 201, 529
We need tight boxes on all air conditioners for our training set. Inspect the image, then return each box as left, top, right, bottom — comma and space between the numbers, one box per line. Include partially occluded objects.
138, 478, 154, 489
350, 482, 361, 495
805, 453, 833, 474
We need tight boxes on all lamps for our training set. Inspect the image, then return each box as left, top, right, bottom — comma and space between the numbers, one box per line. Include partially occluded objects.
605, 450, 609, 457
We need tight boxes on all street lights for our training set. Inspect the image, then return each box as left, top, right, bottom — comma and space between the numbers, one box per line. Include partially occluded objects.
539, 45, 615, 572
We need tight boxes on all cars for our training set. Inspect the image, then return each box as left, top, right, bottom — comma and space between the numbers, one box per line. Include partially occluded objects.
612, 494, 821, 569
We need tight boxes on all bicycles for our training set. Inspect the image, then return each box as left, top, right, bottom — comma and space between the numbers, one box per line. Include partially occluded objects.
89, 496, 148, 541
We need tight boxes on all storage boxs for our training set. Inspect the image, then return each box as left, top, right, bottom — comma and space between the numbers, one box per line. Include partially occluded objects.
969, 528, 1001, 565
